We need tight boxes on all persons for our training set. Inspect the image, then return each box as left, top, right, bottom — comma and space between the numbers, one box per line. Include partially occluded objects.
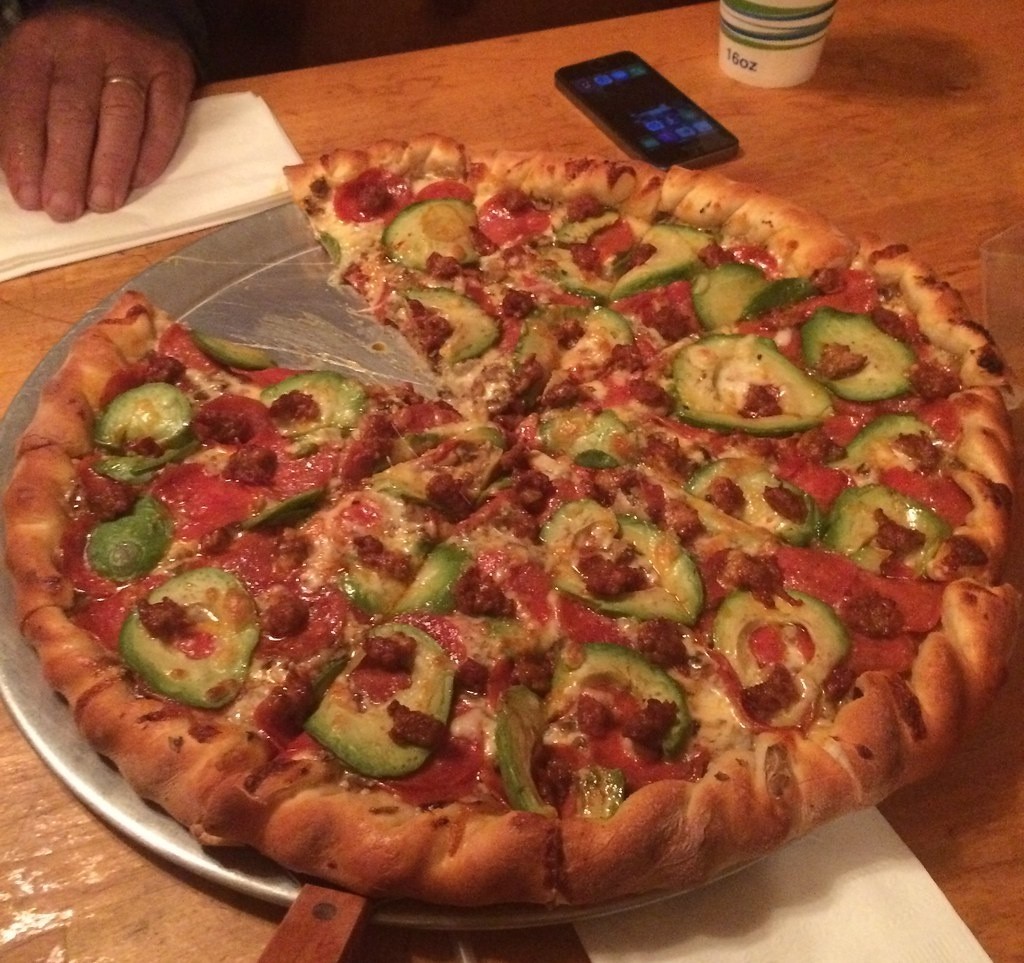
0, 0, 211, 222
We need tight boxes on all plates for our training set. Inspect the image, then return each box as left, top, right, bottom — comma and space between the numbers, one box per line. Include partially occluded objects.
1, 200, 886, 930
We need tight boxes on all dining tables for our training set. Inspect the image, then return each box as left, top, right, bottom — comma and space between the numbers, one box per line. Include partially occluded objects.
0, 0, 1024, 963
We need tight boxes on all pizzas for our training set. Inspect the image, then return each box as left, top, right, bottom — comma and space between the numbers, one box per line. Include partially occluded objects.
0, 132, 1024, 904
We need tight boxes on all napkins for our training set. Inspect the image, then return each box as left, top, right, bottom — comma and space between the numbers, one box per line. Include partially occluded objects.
573, 803, 997, 963
0, 89, 301, 286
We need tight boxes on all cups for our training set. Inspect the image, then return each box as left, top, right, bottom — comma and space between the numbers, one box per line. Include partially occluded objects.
720, 0, 837, 88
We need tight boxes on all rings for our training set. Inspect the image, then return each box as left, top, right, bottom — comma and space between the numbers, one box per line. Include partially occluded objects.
106, 75, 147, 98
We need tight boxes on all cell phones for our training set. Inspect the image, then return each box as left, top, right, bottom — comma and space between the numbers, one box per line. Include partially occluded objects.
554, 51, 739, 170
